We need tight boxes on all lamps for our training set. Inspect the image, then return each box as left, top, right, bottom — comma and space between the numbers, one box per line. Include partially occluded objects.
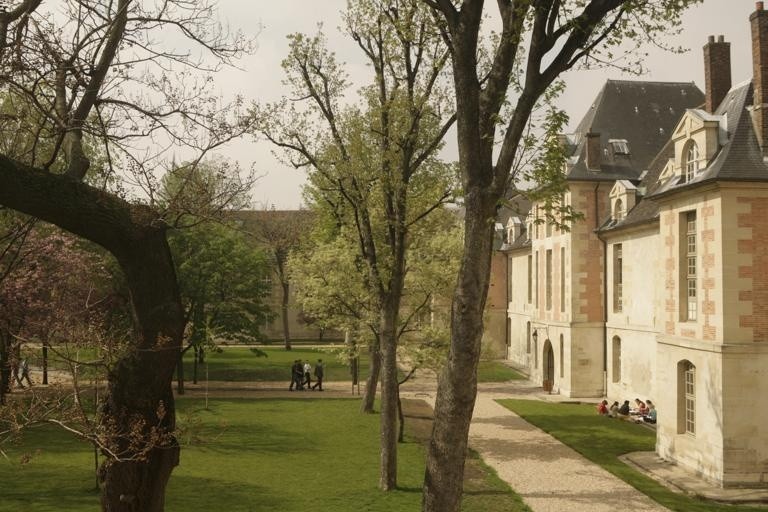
532, 327, 547, 336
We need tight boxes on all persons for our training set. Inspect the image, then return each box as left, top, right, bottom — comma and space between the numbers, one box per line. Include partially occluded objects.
635, 403, 656, 424
597, 399, 608, 416
633, 398, 648, 416
289, 359, 299, 391
638, 400, 652, 416
608, 401, 620, 418
300, 360, 312, 388
616, 400, 631, 416
295, 359, 305, 391
311, 359, 327, 391
